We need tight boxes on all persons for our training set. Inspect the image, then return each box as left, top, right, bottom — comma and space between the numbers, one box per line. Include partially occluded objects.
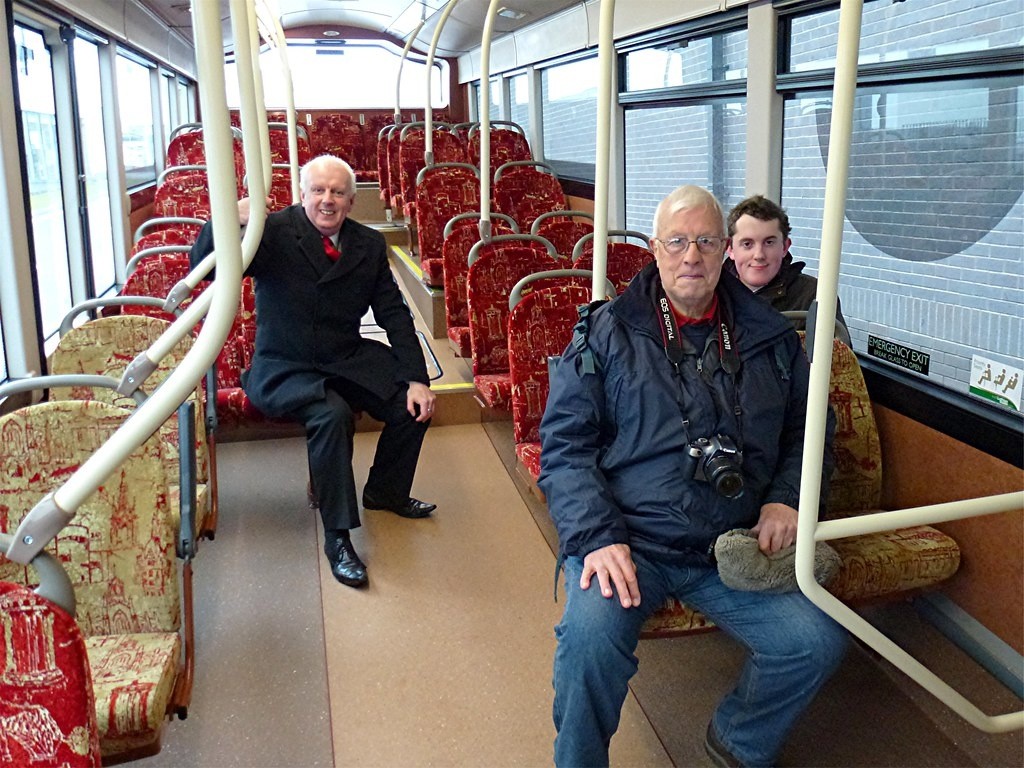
189, 154, 438, 589
537, 183, 853, 768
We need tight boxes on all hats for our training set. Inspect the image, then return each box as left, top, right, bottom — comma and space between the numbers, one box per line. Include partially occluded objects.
714, 528, 843, 594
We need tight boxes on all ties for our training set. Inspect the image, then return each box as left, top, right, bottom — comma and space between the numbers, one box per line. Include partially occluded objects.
322, 237, 341, 260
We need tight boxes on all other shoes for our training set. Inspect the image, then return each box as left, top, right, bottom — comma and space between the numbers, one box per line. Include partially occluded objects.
704, 719, 745, 768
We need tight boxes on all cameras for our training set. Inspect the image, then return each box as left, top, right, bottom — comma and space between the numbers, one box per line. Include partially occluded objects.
679, 433, 746, 499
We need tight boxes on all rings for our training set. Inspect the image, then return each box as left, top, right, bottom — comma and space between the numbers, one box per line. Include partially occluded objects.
427, 409, 431, 411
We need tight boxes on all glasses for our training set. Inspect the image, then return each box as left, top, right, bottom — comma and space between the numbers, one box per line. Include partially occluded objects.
655, 235, 725, 255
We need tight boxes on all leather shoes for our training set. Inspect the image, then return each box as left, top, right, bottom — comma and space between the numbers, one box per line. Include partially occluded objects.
363, 490, 437, 518
324, 537, 368, 587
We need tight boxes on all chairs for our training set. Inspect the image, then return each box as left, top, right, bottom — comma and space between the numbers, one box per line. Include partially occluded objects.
0, 111, 364, 768
364, 114, 959, 640
